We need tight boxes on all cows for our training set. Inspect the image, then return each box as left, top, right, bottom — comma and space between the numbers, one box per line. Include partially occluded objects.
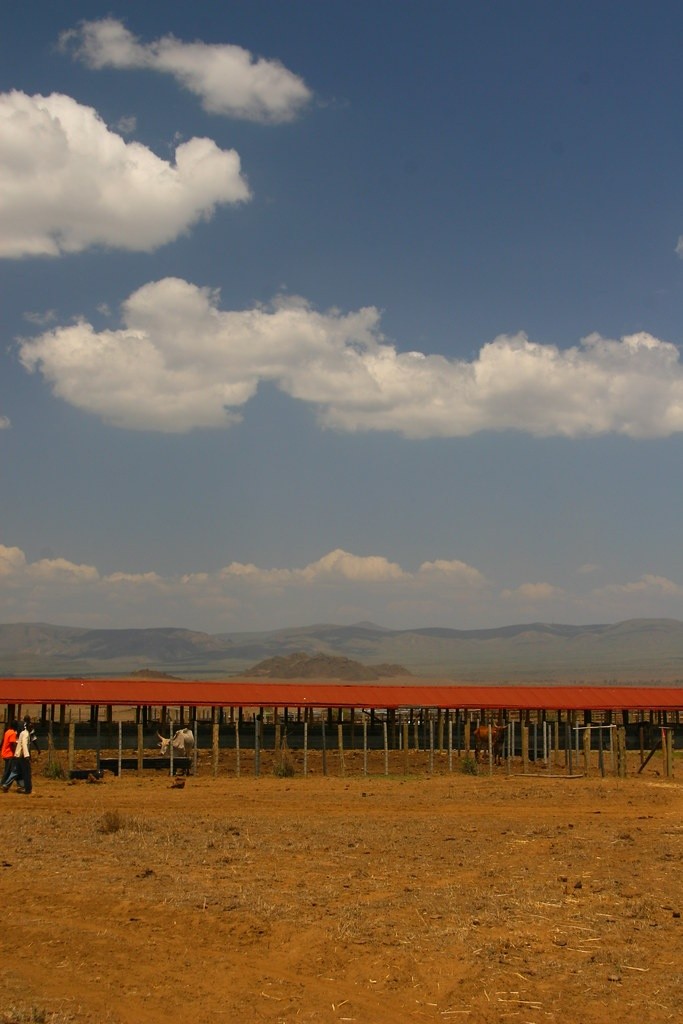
156, 728, 194, 775
473, 718, 509, 766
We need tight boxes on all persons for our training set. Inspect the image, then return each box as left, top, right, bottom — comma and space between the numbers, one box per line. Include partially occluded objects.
14, 722, 32, 794
23, 715, 41, 755
0, 720, 20, 793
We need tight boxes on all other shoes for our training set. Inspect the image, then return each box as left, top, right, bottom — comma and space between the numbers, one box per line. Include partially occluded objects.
24, 790, 32, 794
0, 785, 9, 793
37, 749, 41, 754
3, 784, 9, 792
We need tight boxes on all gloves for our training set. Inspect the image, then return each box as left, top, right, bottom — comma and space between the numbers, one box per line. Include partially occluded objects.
25, 757, 31, 764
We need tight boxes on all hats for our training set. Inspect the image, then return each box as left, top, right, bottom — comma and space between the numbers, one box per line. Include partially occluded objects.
23, 715, 31, 720
